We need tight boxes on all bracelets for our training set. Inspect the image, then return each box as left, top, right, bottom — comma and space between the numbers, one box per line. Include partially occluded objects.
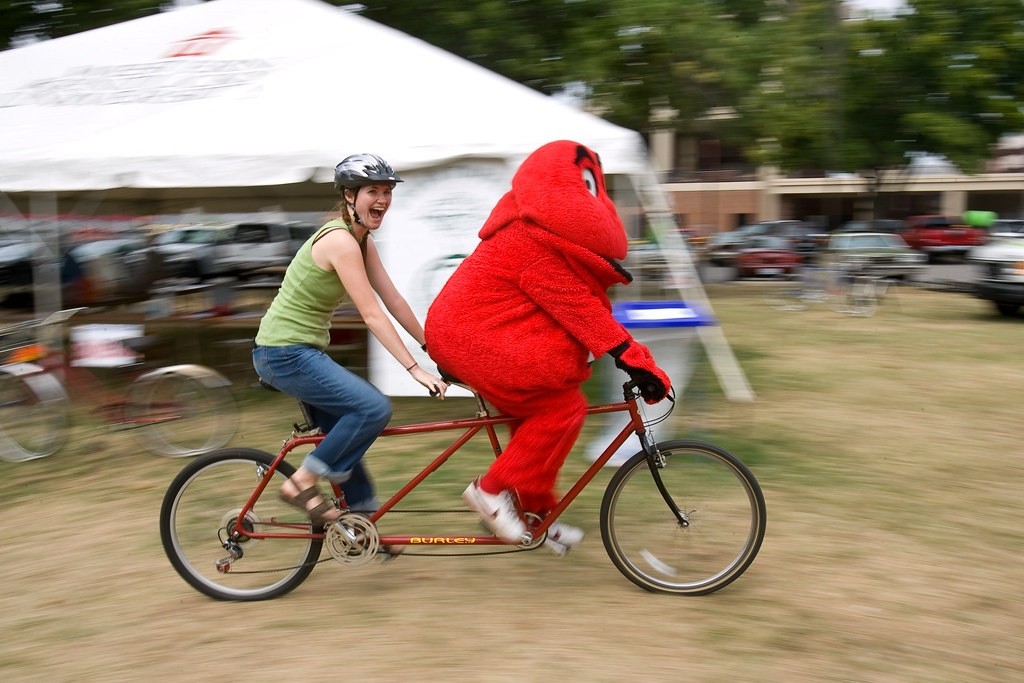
407, 363, 417, 371
421, 344, 426, 352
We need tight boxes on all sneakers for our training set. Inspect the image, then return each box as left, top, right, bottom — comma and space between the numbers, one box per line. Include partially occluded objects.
549, 524, 585, 547
462, 473, 525, 542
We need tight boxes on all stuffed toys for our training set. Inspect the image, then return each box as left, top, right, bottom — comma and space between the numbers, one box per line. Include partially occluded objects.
424, 139, 671, 548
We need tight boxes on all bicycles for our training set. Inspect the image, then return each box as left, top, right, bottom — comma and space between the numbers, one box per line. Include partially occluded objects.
0, 307, 243, 463
156, 359, 770, 598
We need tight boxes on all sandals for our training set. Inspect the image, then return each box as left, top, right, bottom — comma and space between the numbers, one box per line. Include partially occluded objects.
279, 477, 341, 522
377, 545, 406, 559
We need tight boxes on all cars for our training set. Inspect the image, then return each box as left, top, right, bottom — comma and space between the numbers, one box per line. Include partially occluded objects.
0, 211, 319, 308
613, 211, 1024, 320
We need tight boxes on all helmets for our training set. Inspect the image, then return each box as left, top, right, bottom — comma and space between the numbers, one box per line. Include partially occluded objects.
334, 153, 404, 190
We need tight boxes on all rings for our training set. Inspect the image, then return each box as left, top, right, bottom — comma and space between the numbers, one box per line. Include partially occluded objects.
436, 382, 439, 385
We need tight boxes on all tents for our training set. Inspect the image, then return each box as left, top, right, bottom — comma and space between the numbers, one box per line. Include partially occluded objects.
0, 0, 753, 403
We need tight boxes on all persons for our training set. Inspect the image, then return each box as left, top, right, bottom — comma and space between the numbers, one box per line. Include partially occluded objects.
252, 153, 447, 555
